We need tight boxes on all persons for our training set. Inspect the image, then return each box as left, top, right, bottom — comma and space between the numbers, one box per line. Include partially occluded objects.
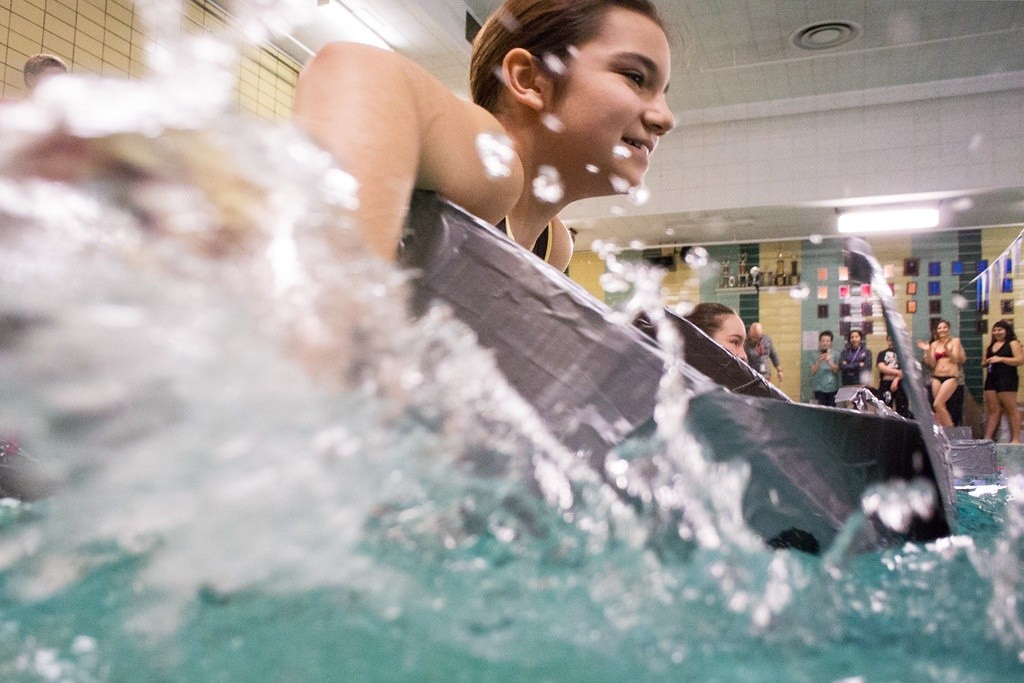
684, 302, 967, 428
293, 0, 676, 407
980, 320, 1024, 444
20, 54, 68, 102
